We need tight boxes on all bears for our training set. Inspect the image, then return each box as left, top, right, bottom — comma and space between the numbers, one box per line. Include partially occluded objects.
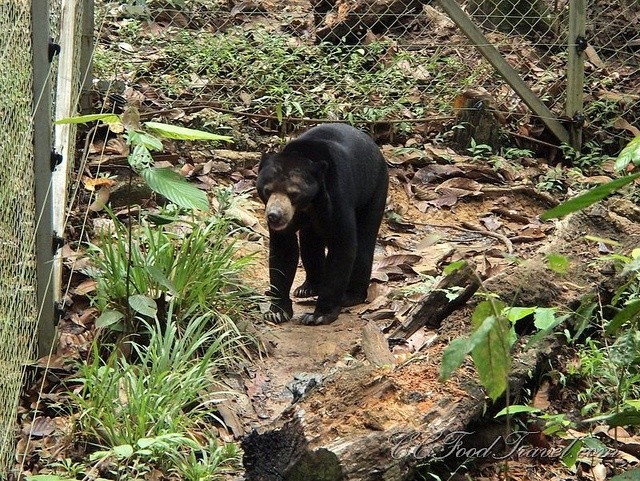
256, 123, 389, 325
